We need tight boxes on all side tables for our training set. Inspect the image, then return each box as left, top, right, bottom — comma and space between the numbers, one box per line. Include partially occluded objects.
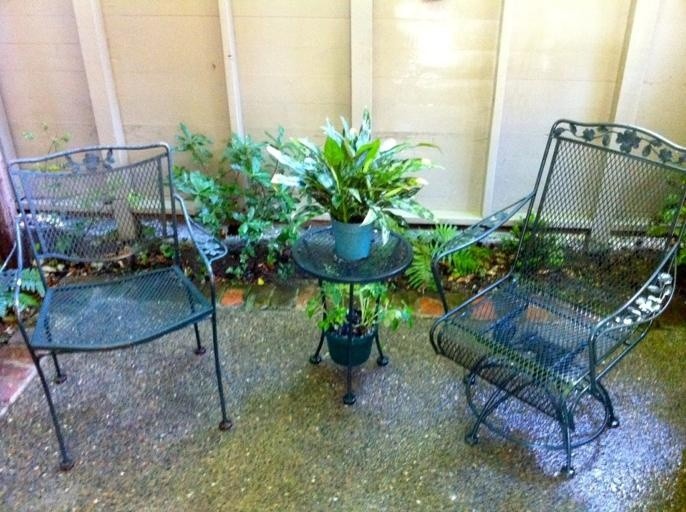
291, 225, 412, 404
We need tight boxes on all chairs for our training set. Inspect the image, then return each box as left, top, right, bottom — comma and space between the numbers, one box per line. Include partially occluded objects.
6, 141, 233, 471
430, 119, 686, 478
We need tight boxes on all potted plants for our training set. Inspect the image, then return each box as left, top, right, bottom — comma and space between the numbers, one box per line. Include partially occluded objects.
263, 106, 445, 262
307, 280, 413, 367
